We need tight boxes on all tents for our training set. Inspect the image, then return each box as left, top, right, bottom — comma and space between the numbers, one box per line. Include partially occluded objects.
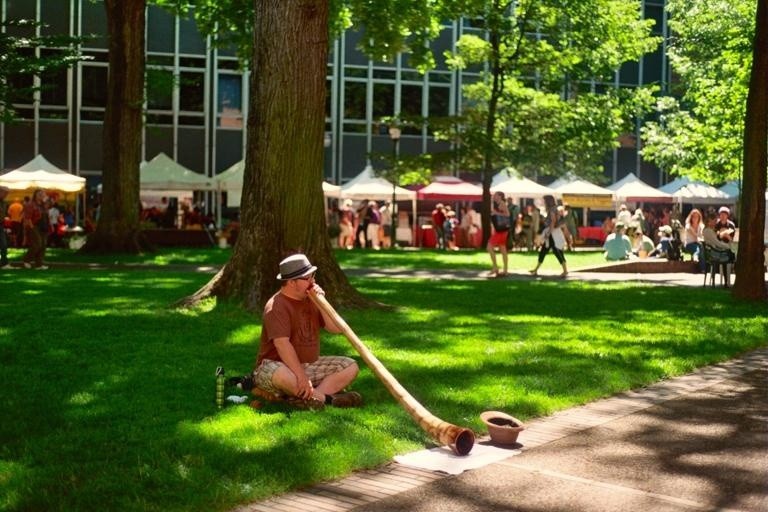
322, 164, 416, 245
548, 170, 612, 226
489, 167, 563, 205
403, 172, 483, 201
658, 175, 739, 210
0, 155, 86, 228
606, 172, 679, 212
138, 152, 244, 225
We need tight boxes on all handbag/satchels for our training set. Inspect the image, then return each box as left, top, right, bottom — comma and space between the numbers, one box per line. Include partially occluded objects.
491, 215, 510, 231
666, 238, 681, 260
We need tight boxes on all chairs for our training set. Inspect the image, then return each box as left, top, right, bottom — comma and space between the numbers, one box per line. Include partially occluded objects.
700, 241, 735, 288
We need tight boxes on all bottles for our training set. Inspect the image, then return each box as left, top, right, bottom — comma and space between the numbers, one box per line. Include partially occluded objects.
214, 367, 227, 411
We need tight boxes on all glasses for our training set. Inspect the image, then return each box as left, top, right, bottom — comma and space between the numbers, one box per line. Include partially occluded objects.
296, 272, 316, 280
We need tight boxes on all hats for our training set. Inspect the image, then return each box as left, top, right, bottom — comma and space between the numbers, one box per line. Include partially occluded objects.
275, 254, 317, 281
658, 225, 671, 235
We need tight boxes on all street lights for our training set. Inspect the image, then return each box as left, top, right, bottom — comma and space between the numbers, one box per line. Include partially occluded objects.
385, 127, 402, 251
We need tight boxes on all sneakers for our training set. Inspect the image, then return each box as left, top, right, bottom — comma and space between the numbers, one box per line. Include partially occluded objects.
0, 264, 14, 270
330, 391, 361, 407
287, 396, 324, 409
35, 265, 48, 271
23, 262, 31, 269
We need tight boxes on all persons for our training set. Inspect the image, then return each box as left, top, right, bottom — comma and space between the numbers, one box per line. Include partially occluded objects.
152, 199, 240, 248
432, 203, 479, 251
487, 192, 577, 279
336, 200, 393, 248
603, 199, 738, 287
251, 250, 364, 411
1, 187, 103, 270
